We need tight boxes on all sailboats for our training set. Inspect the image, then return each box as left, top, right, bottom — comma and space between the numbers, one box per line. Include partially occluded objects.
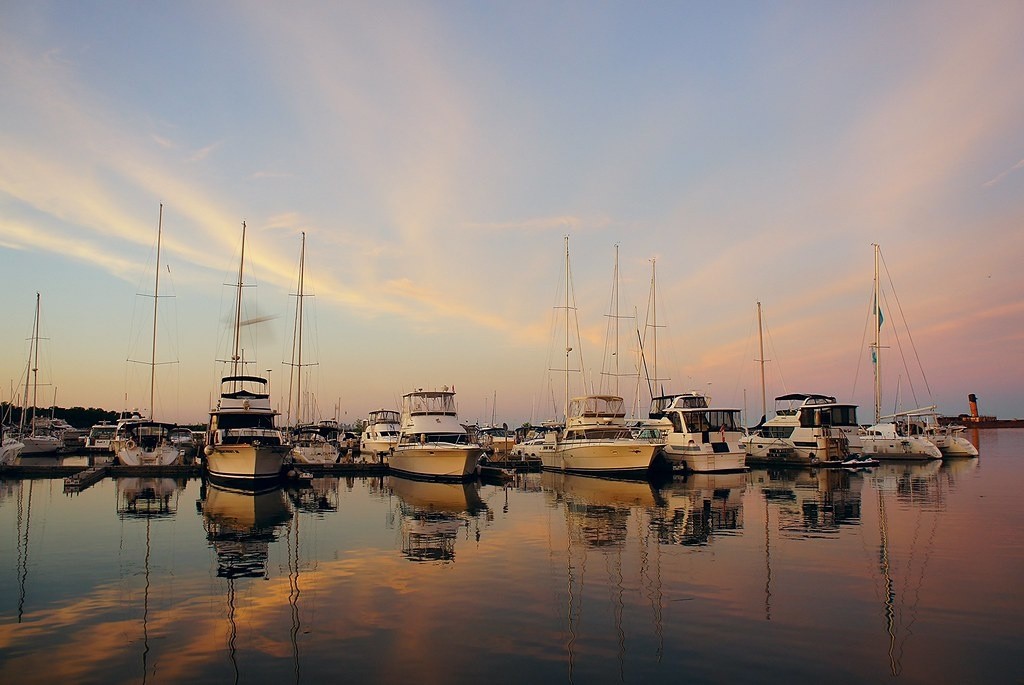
118, 454, 979, 586
0, 206, 981, 488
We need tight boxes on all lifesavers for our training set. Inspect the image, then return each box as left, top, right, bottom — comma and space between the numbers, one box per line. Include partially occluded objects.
126, 439, 135, 450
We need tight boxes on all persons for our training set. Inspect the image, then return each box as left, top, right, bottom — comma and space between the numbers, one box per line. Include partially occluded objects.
388, 444, 395, 456
720, 422, 726, 443
895, 418, 952, 437
